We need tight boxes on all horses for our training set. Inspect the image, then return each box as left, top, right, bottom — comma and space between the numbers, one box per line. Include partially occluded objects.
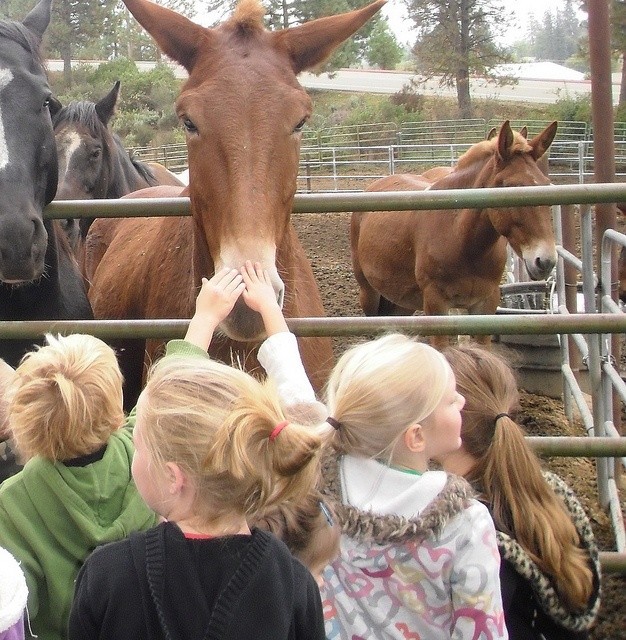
0, 0, 97, 370
39, 80, 192, 265
77, 0, 390, 401
421, 124, 527, 347
348, 119, 557, 353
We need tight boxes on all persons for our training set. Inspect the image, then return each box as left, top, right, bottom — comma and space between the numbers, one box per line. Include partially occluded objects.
239, 259, 510, 639
67, 353, 325, 640
433, 345, 604, 639
255, 489, 342, 588
0, 265, 247, 639
